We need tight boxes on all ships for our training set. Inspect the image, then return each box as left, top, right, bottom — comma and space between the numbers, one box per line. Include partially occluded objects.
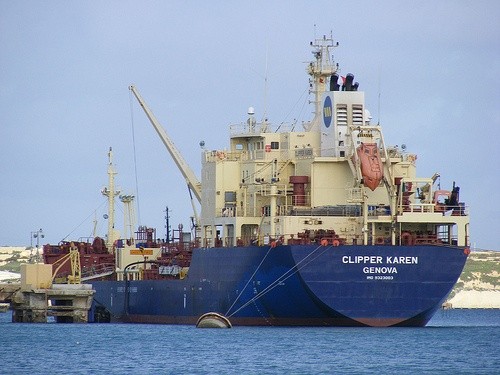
42, 23, 470, 327
